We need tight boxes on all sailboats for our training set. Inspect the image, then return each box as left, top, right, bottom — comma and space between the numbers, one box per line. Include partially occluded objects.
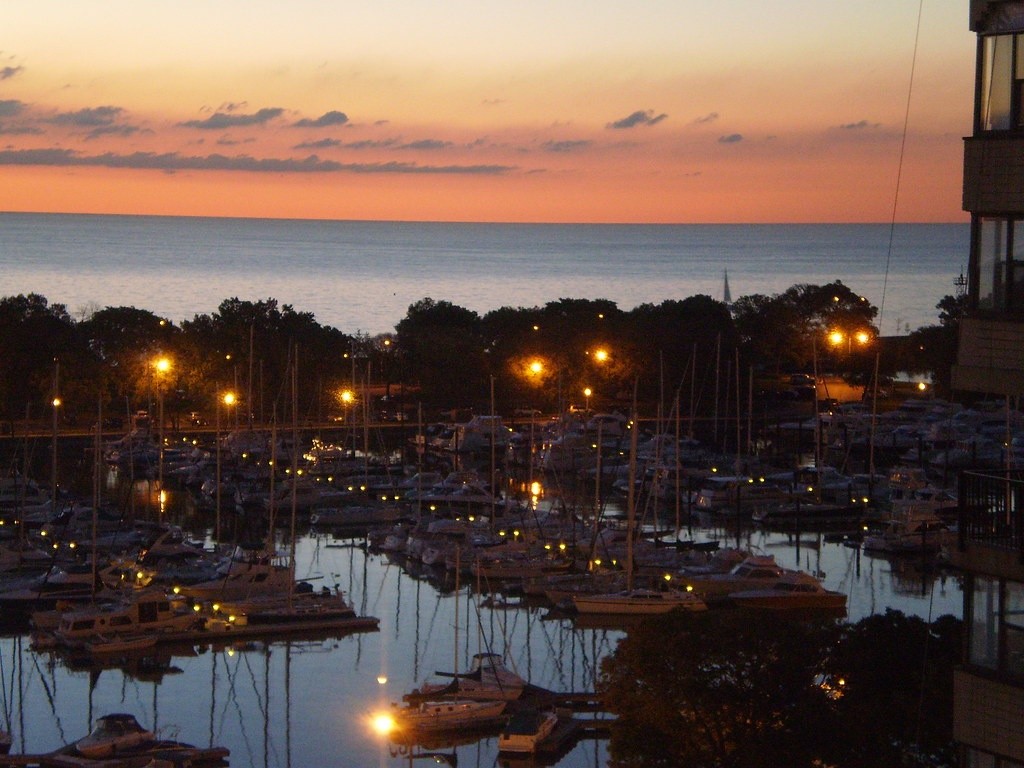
0, 324, 1023, 768
